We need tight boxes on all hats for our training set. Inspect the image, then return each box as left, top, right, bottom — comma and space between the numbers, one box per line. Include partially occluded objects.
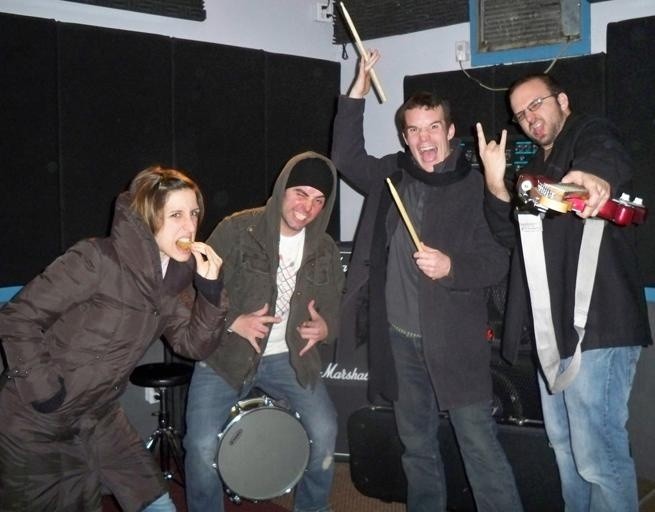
286, 158, 334, 209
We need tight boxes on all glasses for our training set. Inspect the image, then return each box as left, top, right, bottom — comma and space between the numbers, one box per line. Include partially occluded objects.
512, 94, 558, 123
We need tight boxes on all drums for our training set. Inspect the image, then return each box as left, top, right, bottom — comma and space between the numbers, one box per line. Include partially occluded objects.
213, 397, 312, 505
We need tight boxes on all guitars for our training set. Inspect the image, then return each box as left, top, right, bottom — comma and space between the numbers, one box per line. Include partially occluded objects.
524, 180, 649, 226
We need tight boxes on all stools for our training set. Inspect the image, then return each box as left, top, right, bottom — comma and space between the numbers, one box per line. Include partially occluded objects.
128, 362, 192, 481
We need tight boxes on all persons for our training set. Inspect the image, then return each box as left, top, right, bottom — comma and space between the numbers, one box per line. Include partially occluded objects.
0, 167, 232, 512
182, 150, 346, 510
331, 50, 528, 511
476, 74, 652, 510
